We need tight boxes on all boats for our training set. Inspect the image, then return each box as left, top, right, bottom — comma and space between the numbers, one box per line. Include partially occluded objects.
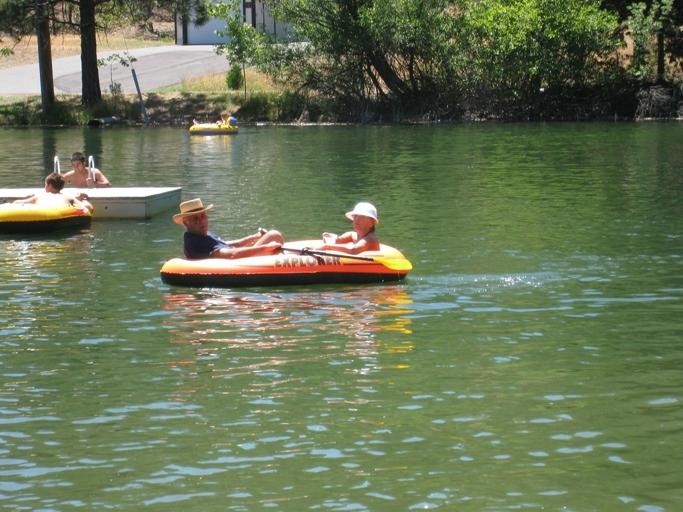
0, 202, 93, 235
158, 236, 413, 288
188, 123, 239, 136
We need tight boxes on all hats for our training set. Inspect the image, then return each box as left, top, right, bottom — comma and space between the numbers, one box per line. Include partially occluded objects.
346, 202, 380, 226
173, 198, 214, 225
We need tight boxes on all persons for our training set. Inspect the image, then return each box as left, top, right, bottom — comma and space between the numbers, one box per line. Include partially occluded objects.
61, 152, 111, 188
192, 111, 227, 125
173, 197, 284, 258
314, 201, 380, 255
13, 172, 92, 215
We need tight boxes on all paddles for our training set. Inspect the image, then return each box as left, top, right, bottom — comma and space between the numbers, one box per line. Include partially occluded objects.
276, 247, 413, 272
